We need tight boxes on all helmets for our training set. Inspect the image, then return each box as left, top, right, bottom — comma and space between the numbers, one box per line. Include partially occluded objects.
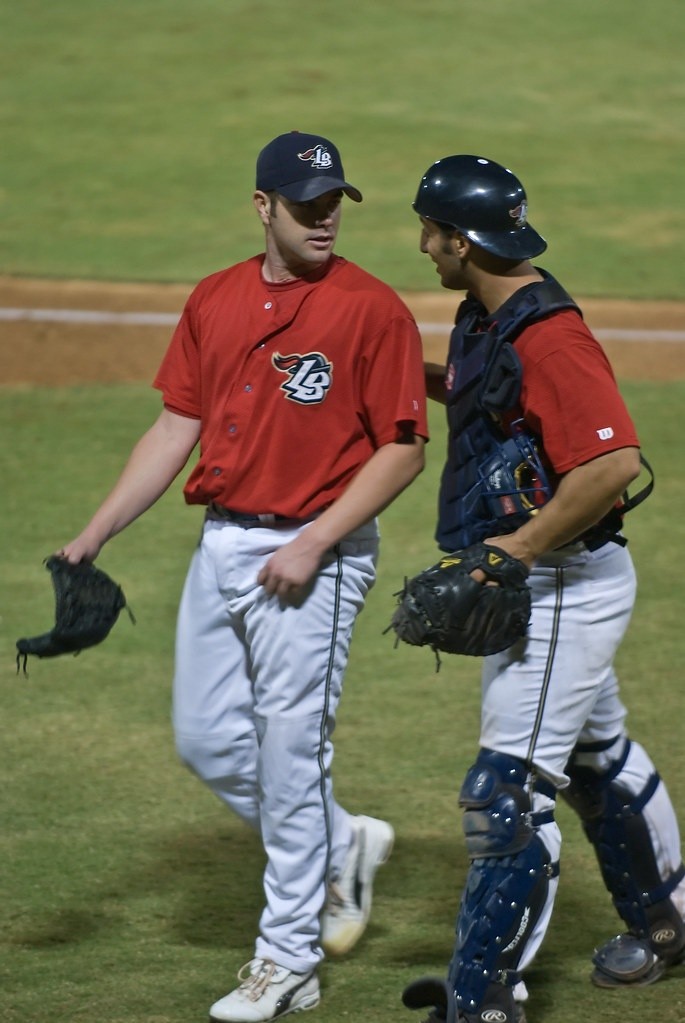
413, 155, 547, 260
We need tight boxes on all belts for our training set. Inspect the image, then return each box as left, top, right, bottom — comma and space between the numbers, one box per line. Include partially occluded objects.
219, 513, 285, 521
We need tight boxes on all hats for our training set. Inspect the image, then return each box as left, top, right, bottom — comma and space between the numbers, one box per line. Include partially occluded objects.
257, 128, 362, 205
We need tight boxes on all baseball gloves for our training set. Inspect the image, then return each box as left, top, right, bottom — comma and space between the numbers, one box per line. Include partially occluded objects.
388, 542, 532, 658
14, 549, 126, 661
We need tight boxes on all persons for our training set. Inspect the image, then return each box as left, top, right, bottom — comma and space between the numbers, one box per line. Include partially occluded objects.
58, 132, 431, 1023
411, 153, 684, 1023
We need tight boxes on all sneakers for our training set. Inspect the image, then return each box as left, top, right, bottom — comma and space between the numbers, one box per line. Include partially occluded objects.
209, 957, 320, 1023
319, 814, 395, 957
590, 950, 685, 987
458, 1006, 528, 1023
